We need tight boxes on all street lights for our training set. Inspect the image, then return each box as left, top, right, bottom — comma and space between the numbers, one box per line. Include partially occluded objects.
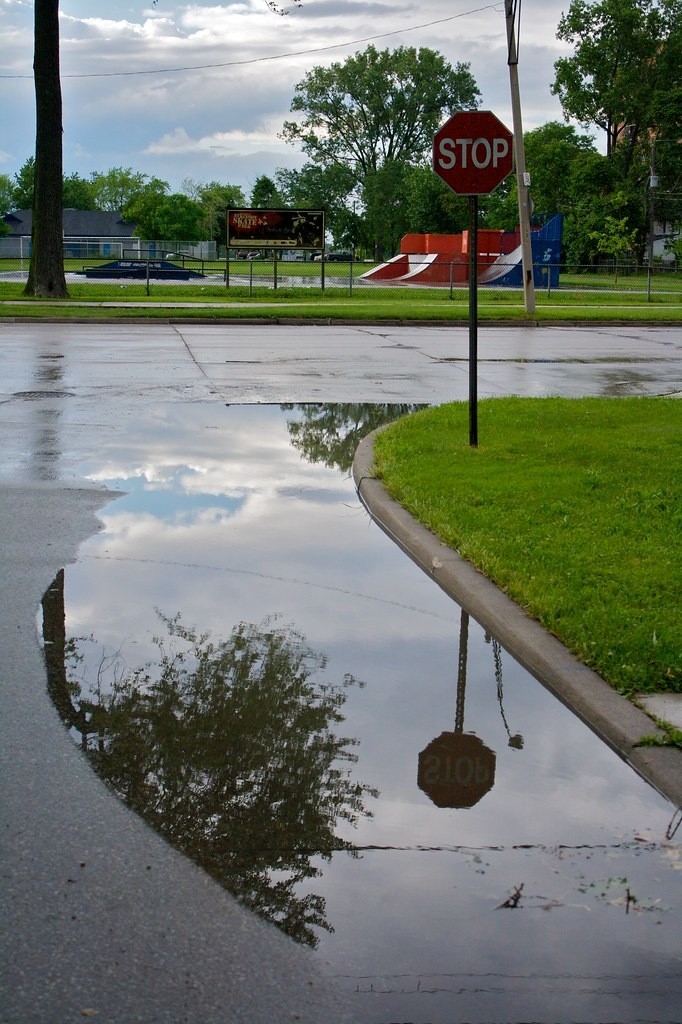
481, 626, 528, 752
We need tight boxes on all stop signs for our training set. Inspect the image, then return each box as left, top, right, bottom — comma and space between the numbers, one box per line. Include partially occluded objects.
415, 731, 497, 810
432, 110, 515, 196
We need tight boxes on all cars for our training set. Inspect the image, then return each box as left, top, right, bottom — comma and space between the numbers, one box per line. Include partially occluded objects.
314, 253, 330, 261
326, 250, 361, 263
246, 252, 260, 260
309, 253, 322, 260
237, 251, 248, 260
